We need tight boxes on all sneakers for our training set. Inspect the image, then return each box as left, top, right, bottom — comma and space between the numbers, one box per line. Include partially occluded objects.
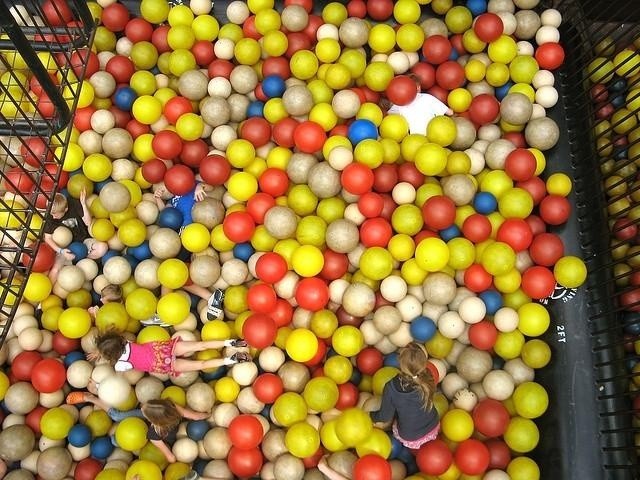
230, 352, 253, 362
206, 288, 226, 321
66, 392, 89, 404
139, 313, 172, 328
231, 339, 247, 347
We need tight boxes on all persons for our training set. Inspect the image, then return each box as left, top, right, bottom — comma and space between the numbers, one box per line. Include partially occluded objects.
138, 171, 225, 325
0, 0, 640, 480
86, 323, 253, 378
316, 453, 351, 480
89, 284, 171, 327
65, 391, 212, 464
371, 340, 441, 456
43, 186, 104, 307
385, 72, 454, 137
127, 470, 266, 480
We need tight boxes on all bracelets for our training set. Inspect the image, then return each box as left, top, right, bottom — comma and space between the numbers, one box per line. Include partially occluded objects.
55, 248, 63, 254
